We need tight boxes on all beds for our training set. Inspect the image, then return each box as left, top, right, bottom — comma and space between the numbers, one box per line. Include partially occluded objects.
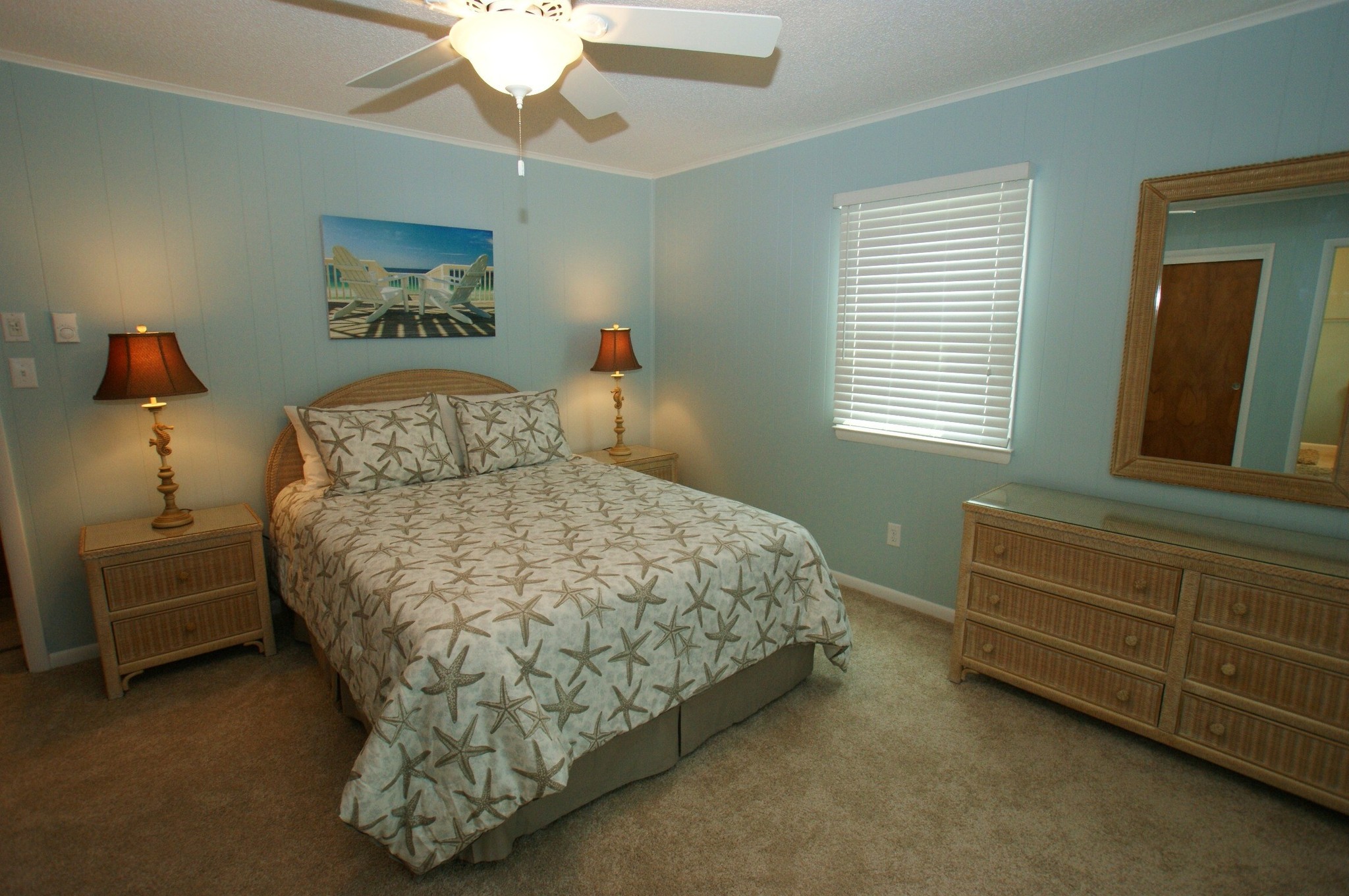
265, 371, 850, 872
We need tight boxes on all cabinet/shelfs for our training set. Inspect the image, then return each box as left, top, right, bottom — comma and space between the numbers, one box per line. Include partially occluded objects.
949, 483, 1349, 814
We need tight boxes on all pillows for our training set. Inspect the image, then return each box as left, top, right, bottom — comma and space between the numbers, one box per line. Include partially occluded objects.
296, 393, 464, 498
283, 398, 426, 491
436, 391, 539, 466
446, 389, 572, 475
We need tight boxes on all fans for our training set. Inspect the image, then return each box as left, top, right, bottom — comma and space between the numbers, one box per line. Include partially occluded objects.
343, 0, 782, 119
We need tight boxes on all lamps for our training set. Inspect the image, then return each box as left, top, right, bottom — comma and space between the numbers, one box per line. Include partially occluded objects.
590, 324, 642, 456
448, 11, 583, 176
93, 325, 208, 527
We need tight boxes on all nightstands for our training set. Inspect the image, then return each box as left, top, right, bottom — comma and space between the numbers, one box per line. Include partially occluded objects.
575, 444, 679, 483
79, 502, 276, 699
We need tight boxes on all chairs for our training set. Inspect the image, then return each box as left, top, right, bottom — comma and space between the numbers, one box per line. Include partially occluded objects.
416, 254, 492, 324
331, 245, 413, 323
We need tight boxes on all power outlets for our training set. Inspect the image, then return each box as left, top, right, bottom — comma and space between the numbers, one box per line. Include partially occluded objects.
1, 312, 30, 342
887, 522, 901, 547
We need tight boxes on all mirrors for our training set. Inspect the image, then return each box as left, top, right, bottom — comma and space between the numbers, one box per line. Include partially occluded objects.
1109, 150, 1349, 510
